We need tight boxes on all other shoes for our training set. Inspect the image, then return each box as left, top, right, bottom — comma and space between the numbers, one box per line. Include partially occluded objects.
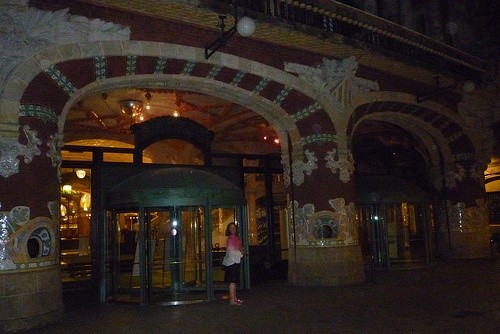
231, 299, 243, 305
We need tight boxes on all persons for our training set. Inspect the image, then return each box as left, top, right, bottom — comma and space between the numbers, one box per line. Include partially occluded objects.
220, 222, 246, 306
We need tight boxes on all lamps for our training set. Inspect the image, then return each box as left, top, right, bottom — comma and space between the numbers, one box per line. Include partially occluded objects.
417, 76, 474, 104
205, 15, 255, 60
